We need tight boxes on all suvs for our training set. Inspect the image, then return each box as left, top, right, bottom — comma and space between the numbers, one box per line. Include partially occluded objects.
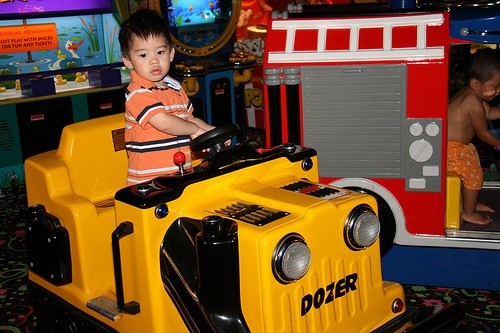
24, 112, 415, 333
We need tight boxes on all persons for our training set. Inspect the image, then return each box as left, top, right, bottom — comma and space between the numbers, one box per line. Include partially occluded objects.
118, 8, 231, 189
447, 46, 500, 224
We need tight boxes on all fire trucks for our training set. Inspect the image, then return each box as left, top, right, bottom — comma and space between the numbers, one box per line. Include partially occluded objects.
262, 8, 500, 291
